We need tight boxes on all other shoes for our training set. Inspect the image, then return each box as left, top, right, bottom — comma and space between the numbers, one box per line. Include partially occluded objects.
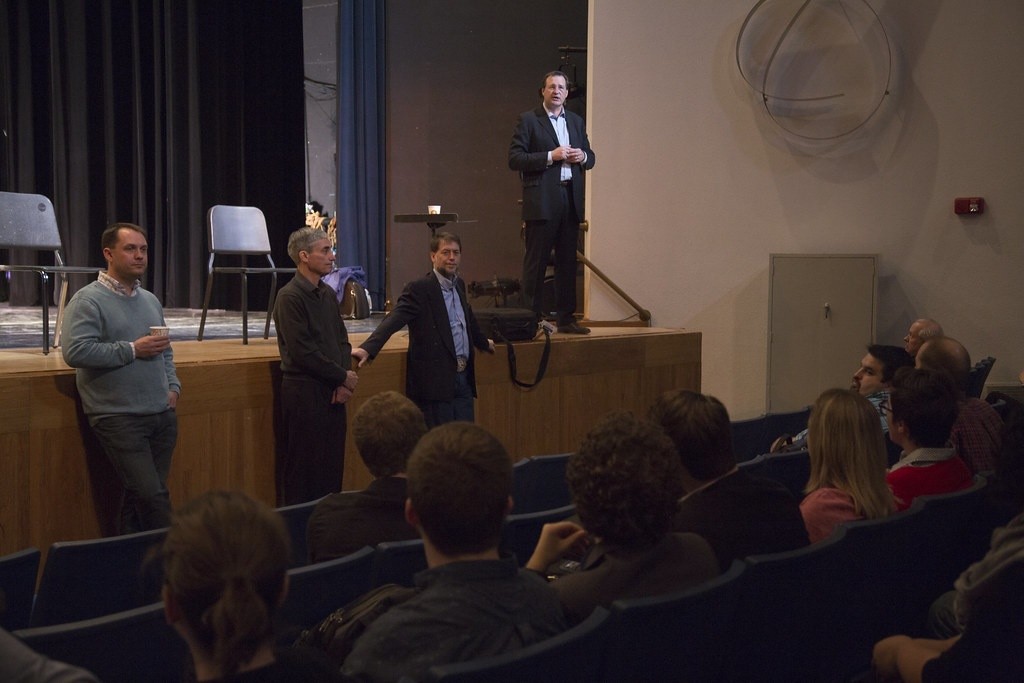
557, 321, 591, 334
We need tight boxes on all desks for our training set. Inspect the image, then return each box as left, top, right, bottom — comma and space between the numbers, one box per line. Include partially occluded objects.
394, 212, 477, 271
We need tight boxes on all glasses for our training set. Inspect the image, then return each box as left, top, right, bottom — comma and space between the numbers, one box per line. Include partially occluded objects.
879, 400, 894, 416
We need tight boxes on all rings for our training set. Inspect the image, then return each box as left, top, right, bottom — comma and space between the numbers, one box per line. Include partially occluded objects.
577, 156, 579, 159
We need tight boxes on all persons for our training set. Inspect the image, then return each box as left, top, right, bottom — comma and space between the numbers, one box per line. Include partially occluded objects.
904, 318, 944, 356
304, 391, 428, 560
798, 388, 897, 543
916, 337, 1007, 471
771, 344, 915, 452
145, 491, 355, 682
508, 71, 595, 334
342, 421, 573, 682
878, 367, 973, 511
274, 227, 359, 563
651, 388, 811, 573
0, 627, 97, 683
868, 400, 1024, 683
526, 408, 720, 619
62, 223, 182, 531
351, 232, 494, 428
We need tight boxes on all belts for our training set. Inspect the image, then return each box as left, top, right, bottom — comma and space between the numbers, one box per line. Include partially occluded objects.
561, 180, 572, 187
457, 358, 470, 372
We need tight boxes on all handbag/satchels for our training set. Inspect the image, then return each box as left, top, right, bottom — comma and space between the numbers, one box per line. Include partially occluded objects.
772, 433, 807, 454
293, 583, 417, 669
338, 278, 370, 320
474, 308, 551, 387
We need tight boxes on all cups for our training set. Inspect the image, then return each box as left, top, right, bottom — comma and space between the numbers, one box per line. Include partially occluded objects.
428, 206, 441, 214
150, 326, 169, 338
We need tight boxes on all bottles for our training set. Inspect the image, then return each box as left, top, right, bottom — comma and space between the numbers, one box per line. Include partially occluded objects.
385, 300, 392, 315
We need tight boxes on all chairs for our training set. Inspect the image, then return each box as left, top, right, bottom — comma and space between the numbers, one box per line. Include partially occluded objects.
0, 191, 107, 356
0, 392, 1024, 683
196, 205, 295, 347
